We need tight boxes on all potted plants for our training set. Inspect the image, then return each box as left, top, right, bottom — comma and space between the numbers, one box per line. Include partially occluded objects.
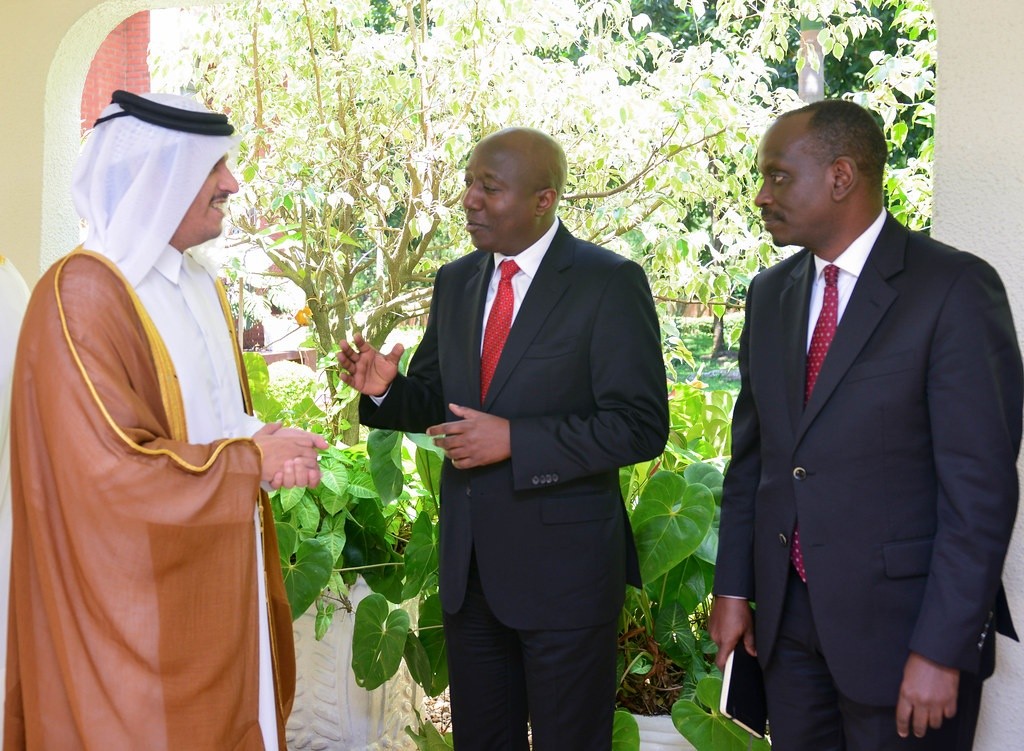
269, 442, 423, 751
609, 467, 770, 750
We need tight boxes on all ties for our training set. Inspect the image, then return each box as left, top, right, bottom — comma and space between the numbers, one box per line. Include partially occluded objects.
481, 260, 519, 403
790, 265, 844, 583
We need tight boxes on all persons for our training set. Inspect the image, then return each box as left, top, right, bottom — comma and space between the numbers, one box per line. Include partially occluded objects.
2, 86, 332, 751
330, 125, 672, 751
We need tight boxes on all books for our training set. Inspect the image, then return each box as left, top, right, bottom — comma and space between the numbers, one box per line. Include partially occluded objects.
705, 97, 1024, 750
717, 603, 770, 740
